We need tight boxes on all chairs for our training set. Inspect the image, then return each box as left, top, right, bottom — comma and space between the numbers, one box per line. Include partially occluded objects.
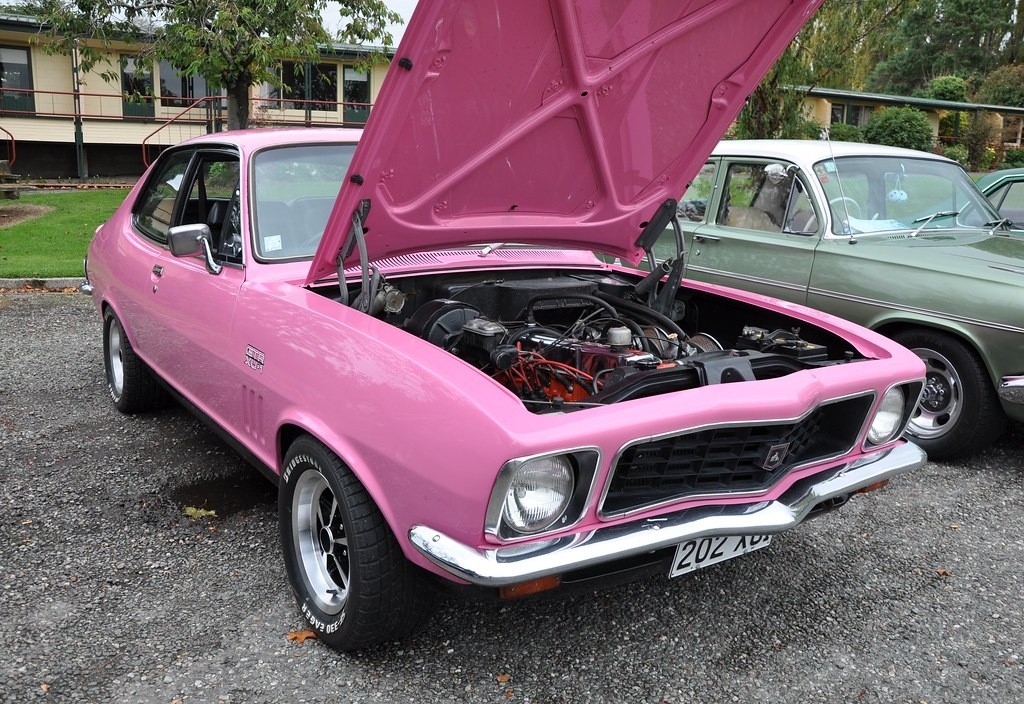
789, 207, 819, 232
205, 200, 307, 263
725, 206, 779, 233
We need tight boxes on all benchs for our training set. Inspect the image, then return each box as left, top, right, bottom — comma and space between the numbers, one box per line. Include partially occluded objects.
140, 197, 339, 259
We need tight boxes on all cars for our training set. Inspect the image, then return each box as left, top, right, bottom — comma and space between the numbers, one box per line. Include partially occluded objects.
597, 139, 1024, 463
888, 168, 1024, 233
82, 0, 927, 647
